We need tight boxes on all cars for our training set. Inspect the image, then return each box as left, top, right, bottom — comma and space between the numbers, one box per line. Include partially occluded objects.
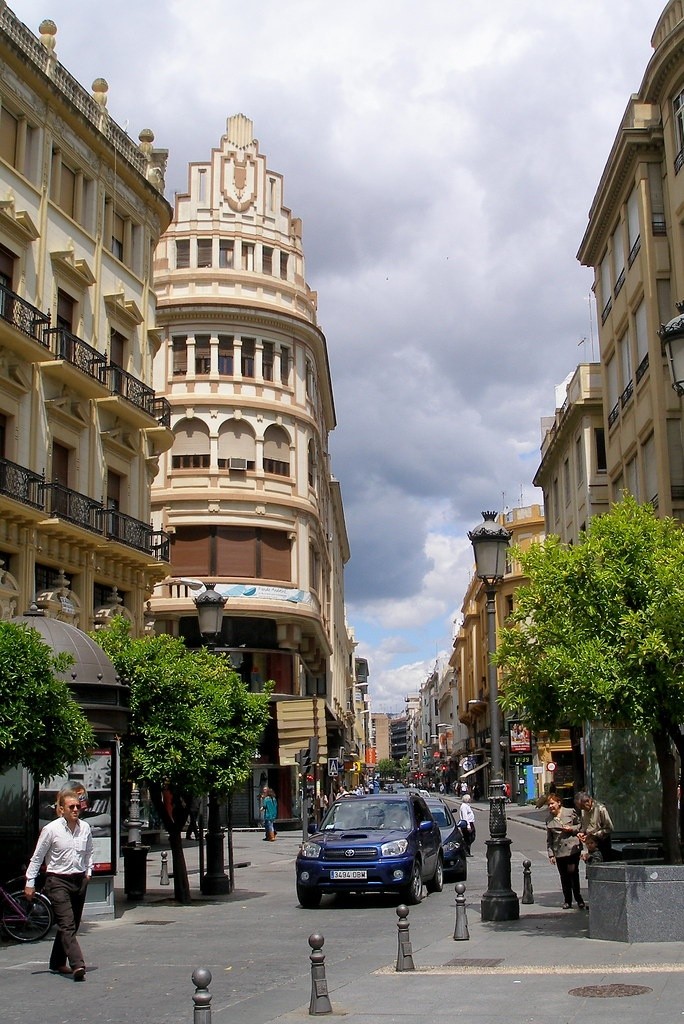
377, 803, 467, 881
384, 783, 458, 813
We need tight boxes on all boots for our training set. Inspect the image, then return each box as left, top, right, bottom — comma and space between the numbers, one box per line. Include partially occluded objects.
269, 832, 275, 841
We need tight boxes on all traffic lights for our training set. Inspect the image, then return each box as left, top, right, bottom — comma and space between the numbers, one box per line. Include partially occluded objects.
414, 774, 418, 778
301, 749, 312, 774
442, 766, 446, 776
420, 772, 424, 776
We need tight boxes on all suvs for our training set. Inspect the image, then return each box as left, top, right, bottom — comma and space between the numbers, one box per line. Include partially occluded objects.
296, 791, 444, 907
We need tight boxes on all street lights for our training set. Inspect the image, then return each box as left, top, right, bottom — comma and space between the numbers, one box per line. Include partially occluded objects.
192, 582, 231, 896
467, 510, 519, 922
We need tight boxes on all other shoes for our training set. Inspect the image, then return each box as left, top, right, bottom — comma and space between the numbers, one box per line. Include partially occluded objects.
49, 966, 73, 973
263, 838, 269, 840
563, 903, 573, 908
274, 831, 277, 834
73, 968, 86, 981
186, 836, 194, 840
196, 837, 199, 841
579, 902, 585, 910
470, 854, 474, 857
466, 852, 470, 857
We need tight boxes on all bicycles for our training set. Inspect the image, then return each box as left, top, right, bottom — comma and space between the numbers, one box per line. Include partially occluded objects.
0, 886, 54, 942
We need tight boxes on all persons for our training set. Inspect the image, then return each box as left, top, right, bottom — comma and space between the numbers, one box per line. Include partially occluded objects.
260, 787, 277, 841
307, 772, 480, 829
24, 790, 94, 982
578, 834, 605, 910
575, 791, 614, 851
38, 780, 113, 837
263, 787, 278, 842
545, 792, 585, 909
185, 795, 202, 840
458, 794, 476, 857
509, 723, 530, 747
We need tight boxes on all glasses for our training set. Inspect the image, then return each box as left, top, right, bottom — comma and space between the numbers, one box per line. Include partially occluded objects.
60, 805, 81, 809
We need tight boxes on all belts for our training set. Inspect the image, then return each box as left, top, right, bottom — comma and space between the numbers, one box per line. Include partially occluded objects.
57, 875, 79, 881
